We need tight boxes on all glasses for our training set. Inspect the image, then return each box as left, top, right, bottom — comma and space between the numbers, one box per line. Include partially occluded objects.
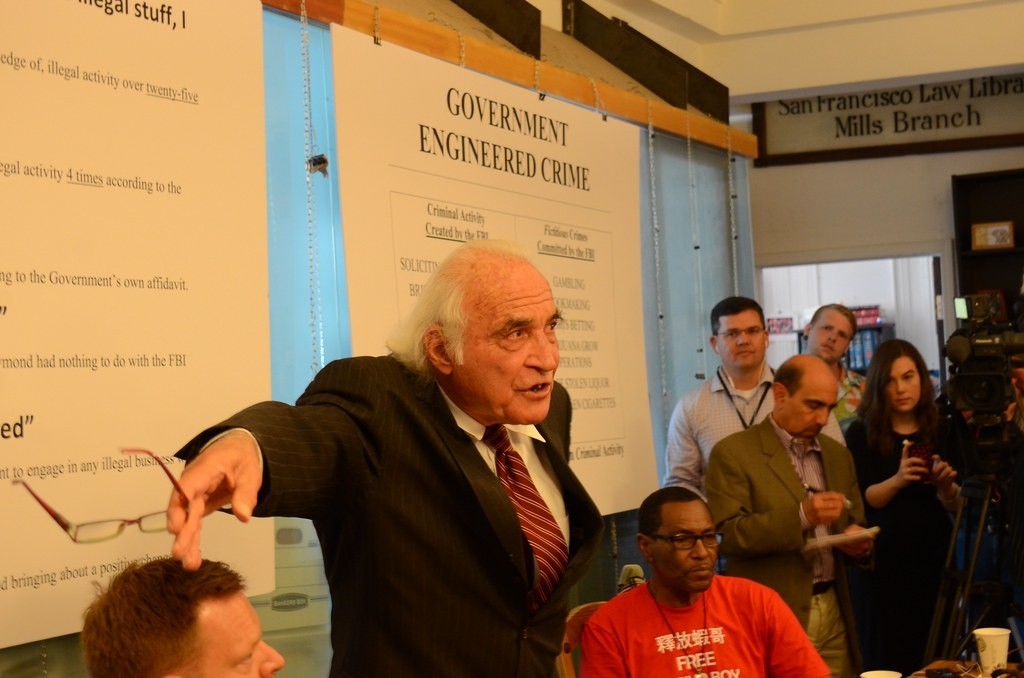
719, 327, 764, 338
651, 533, 724, 549
12, 448, 189, 543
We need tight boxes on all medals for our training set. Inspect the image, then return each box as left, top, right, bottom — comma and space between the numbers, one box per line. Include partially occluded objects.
697, 674, 704, 678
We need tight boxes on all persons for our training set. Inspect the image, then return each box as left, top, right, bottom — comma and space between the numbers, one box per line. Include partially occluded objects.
166, 240, 604, 678
843, 339, 974, 678
579, 486, 834, 678
663, 296, 779, 503
1007, 617, 1024, 663
705, 355, 875, 678
81, 556, 286, 678
804, 304, 868, 436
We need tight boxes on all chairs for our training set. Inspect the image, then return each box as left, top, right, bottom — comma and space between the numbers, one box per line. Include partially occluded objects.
553, 601, 607, 678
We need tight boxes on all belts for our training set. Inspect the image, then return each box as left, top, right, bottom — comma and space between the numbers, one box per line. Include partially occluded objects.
812, 580, 837, 595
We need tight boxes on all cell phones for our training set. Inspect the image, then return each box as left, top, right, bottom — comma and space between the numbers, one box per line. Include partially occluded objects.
909, 443, 935, 482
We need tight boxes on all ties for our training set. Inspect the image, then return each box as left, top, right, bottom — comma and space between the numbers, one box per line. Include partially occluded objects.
482, 423, 570, 617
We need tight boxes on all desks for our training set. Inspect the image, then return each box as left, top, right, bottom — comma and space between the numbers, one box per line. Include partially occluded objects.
904, 660, 1024, 678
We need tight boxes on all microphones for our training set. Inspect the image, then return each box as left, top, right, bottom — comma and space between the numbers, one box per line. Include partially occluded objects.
946, 336, 971, 363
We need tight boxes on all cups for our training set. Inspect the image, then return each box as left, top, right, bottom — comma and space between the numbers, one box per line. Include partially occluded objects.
860, 670, 902, 678
973, 628, 1011, 675
